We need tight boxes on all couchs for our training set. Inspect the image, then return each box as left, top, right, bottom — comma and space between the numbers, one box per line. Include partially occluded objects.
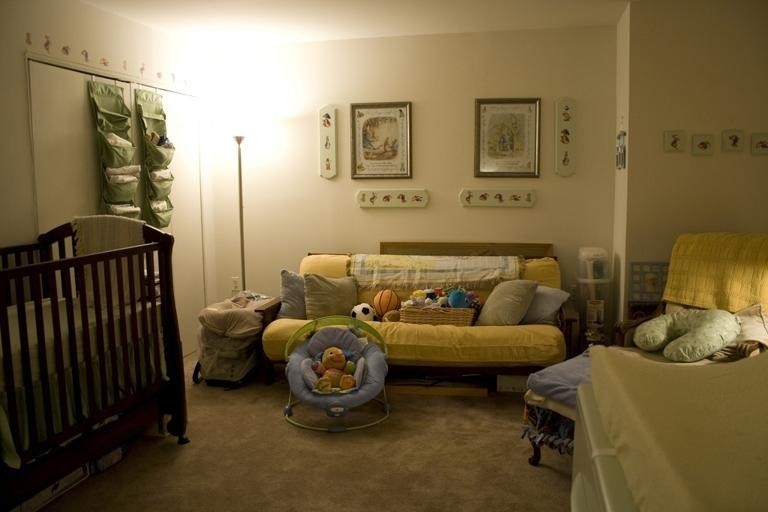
262, 252, 568, 398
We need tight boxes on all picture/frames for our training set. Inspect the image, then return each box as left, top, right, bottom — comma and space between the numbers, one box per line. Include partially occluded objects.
473, 96, 540, 178
349, 102, 413, 178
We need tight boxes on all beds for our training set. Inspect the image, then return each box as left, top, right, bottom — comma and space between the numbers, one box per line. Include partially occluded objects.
570, 346, 768, 511
0, 214, 190, 510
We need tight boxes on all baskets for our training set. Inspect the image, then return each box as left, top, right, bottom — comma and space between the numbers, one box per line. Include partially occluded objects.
399, 305, 477, 327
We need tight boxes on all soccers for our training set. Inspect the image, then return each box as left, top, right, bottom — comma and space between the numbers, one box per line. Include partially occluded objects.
351, 303, 377, 322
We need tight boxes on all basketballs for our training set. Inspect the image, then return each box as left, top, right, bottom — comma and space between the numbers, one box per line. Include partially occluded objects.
374, 289, 400, 317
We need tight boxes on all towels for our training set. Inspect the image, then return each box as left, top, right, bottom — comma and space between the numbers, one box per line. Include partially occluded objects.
72, 213, 146, 307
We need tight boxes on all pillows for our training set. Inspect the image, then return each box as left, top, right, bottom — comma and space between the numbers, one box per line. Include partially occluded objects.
633, 309, 740, 363
520, 285, 571, 325
473, 276, 538, 326
276, 269, 306, 318
304, 272, 358, 320
713, 303, 768, 362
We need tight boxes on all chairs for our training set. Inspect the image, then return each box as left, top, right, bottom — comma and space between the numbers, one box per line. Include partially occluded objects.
614, 232, 768, 359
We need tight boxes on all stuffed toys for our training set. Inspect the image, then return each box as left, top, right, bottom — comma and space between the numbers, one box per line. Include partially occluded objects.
312, 347, 357, 392
351, 280, 482, 321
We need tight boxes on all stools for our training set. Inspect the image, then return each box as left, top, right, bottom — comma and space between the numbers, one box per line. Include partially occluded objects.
520, 348, 591, 466
